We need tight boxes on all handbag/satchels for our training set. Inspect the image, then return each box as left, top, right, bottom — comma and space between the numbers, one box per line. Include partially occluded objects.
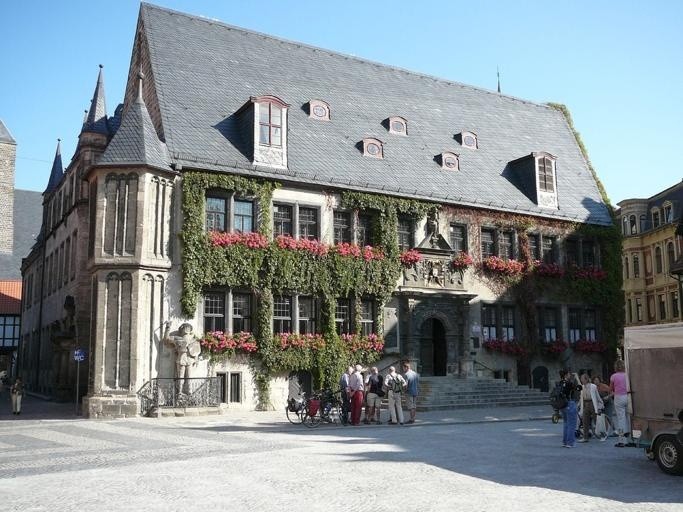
287, 398, 302, 411
376, 388, 385, 397
309, 400, 320, 416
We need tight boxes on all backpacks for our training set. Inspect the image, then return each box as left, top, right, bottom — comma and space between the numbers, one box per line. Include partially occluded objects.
550, 381, 568, 409
390, 373, 402, 392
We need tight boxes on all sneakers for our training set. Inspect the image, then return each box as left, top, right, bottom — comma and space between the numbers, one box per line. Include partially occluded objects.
562, 432, 630, 448
361, 418, 415, 425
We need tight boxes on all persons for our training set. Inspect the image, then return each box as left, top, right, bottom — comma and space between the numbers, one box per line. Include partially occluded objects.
557, 367, 581, 448
340, 364, 406, 426
10, 378, 25, 415
610, 359, 636, 447
592, 376, 614, 432
402, 363, 419, 423
164, 321, 201, 396
577, 374, 608, 443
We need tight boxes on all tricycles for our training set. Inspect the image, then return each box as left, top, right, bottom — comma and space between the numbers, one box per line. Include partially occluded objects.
613, 322, 682, 476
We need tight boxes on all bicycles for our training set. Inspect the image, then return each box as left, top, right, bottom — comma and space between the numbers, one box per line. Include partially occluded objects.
286, 384, 349, 428
570, 410, 612, 441
550, 409, 560, 424
